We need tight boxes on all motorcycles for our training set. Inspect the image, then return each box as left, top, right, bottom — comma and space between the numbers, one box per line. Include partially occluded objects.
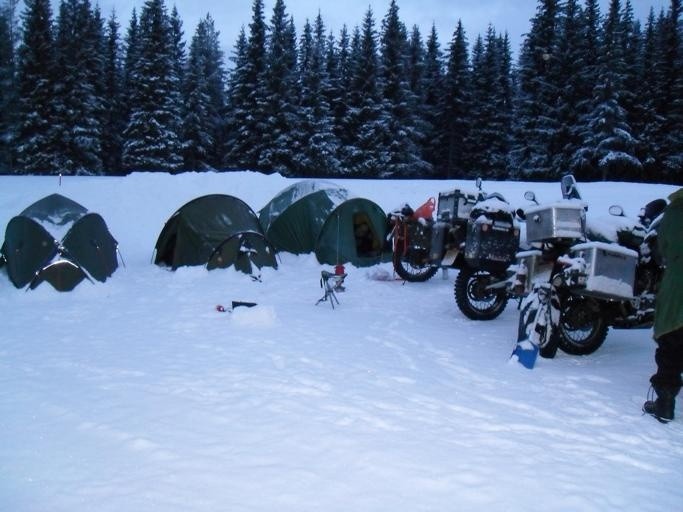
514, 174, 670, 358
454, 190, 553, 320
386, 175, 509, 282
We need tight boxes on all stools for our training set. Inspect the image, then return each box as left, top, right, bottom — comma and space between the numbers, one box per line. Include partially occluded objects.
315, 270, 347, 309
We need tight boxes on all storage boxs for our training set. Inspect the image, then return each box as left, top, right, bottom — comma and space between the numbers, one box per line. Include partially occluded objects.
573, 247, 637, 301
526, 208, 585, 242
437, 190, 480, 222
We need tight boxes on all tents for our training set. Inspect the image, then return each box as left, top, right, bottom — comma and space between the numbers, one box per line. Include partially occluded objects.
152, 193, 279, 271
257, 179, 393, 269
1, 194, 121, 293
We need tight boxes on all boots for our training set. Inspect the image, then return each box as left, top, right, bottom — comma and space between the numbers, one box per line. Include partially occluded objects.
644, 374, 683, 424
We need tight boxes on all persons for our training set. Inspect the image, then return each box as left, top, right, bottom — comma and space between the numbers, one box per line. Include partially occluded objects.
353, 221, 370, 257
640, 187, 683, 421
